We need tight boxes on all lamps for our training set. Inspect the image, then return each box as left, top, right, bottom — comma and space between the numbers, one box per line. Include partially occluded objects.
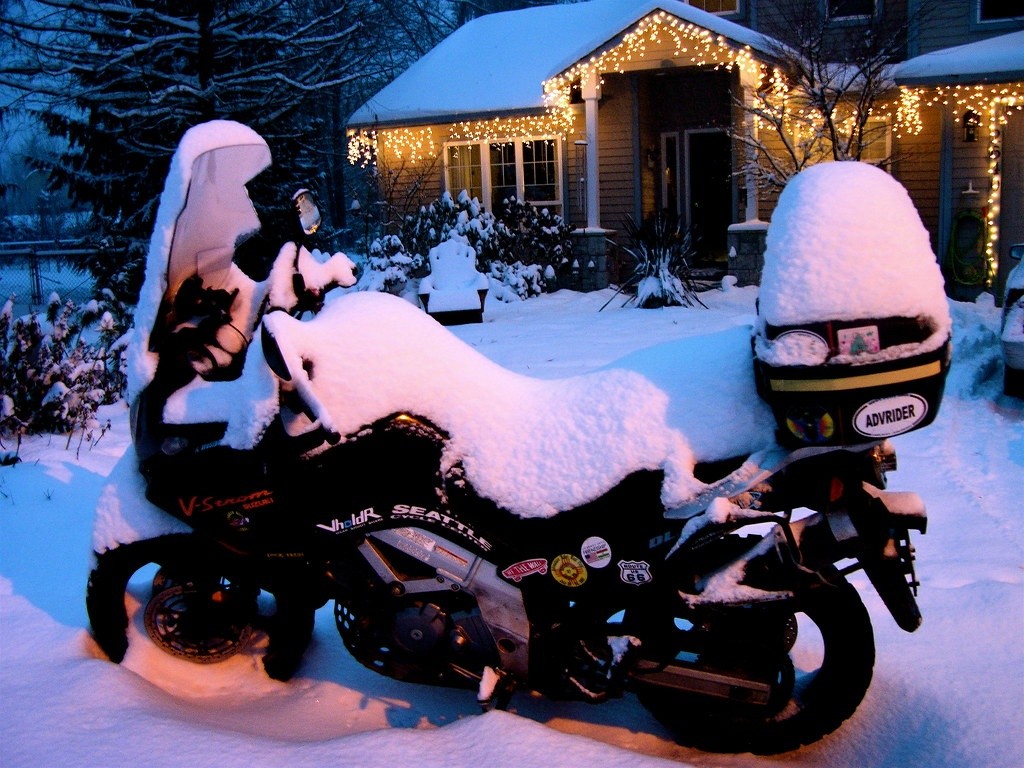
963, 110, 980, 143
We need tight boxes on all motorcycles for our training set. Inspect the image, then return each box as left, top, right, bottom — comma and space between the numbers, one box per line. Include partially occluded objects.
85, 139, 948, 755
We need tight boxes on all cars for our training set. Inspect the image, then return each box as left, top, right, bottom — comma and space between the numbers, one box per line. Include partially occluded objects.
1001, 241, 1024, 402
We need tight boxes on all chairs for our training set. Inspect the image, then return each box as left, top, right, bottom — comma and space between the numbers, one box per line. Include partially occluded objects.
418, 240, 489, 325
